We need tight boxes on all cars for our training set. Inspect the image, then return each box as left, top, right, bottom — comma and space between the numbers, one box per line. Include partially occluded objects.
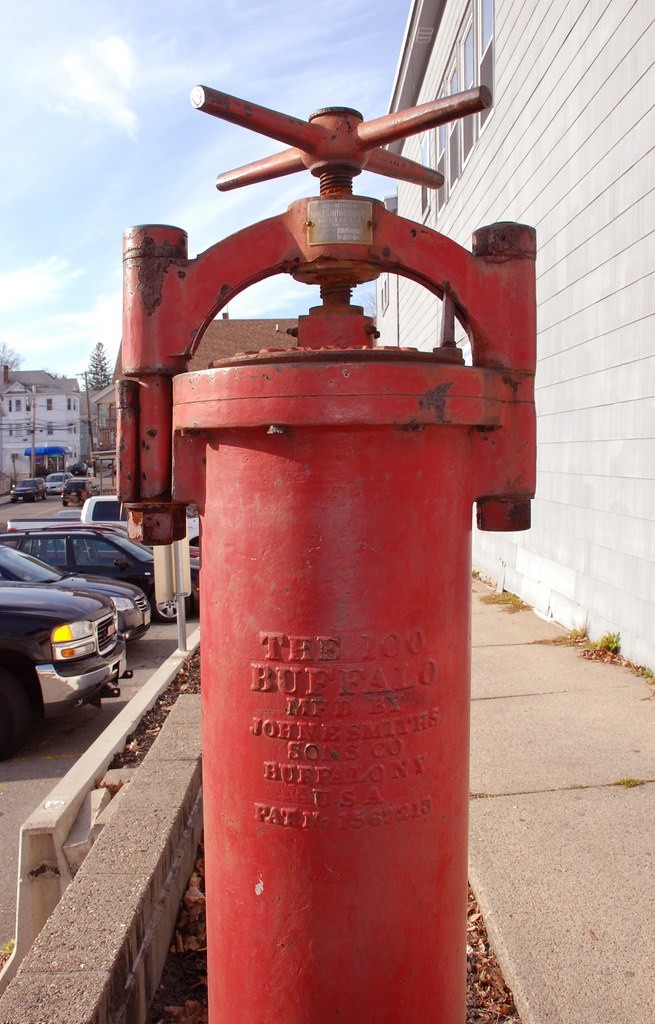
10, 478, 47, 503
0, 495, 199, 763
45, 463, 100, 506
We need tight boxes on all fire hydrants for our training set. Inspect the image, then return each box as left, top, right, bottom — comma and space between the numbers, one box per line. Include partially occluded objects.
113, 85, 537, 1024
